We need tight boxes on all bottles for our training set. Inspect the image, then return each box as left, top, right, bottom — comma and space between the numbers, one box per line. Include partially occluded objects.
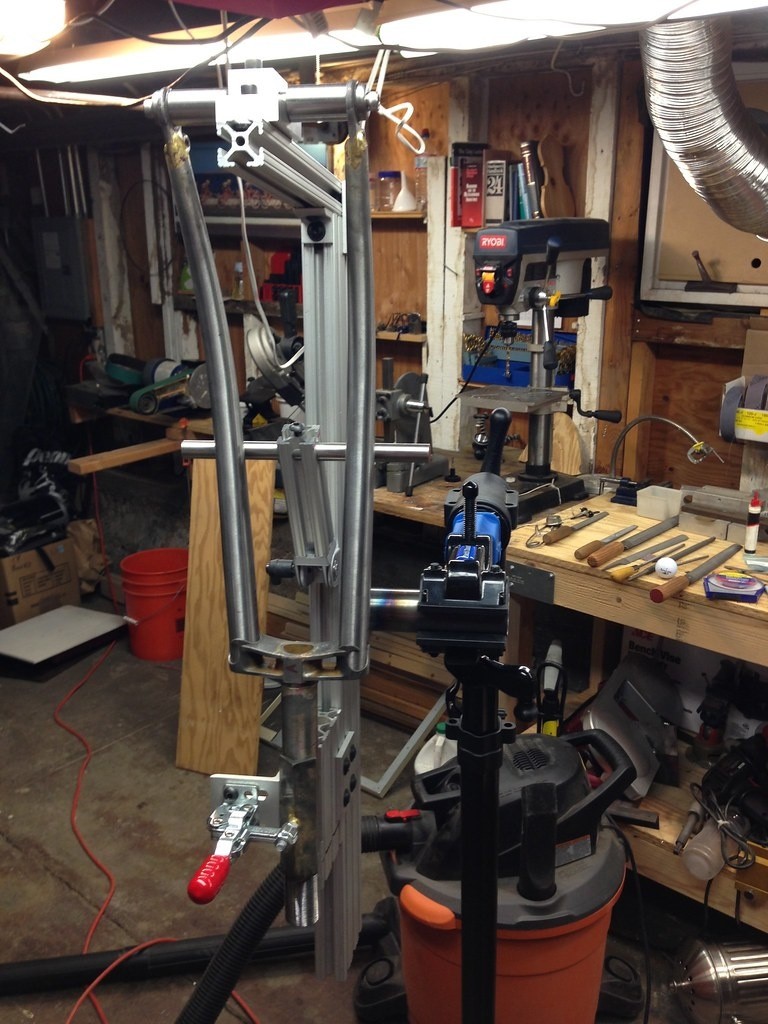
414, 127, 437, 211
376, 170, 401, 211
413, 722, 458, 776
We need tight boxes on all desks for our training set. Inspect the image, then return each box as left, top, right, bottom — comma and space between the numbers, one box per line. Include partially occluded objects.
369, 447, 577, 529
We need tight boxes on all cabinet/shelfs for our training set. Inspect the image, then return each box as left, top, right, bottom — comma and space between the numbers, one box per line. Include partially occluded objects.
167, 209, 426, 439
486, 488, 768, 933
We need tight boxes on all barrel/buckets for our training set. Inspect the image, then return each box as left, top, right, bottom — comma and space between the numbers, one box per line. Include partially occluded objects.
397, 825, 627, 1024
119, 547, 189, 661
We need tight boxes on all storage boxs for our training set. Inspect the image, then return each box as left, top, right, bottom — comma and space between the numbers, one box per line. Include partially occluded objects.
447, 141, 509, 232
0, 538, 83, 631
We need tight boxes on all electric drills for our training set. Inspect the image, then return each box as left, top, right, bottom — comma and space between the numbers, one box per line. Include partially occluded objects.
444, 217, 622, 528
700, 732, 768, 841
